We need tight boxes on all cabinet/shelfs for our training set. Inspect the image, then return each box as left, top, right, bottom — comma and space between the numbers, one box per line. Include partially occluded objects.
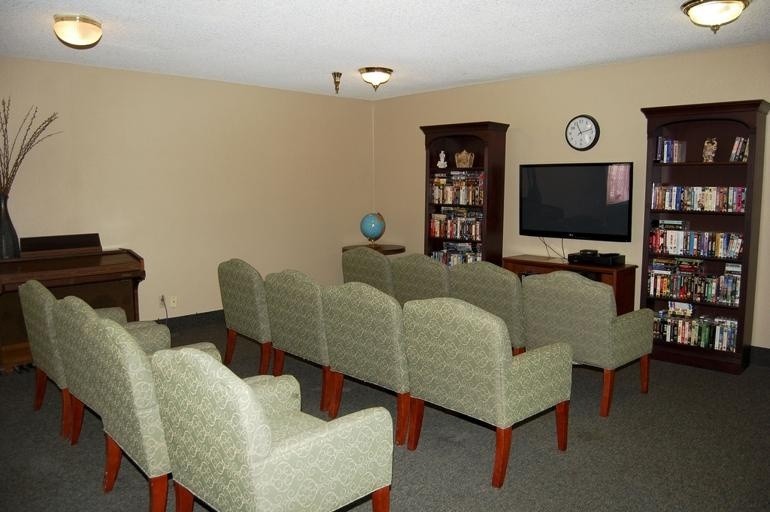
638, 97, 770, 374
419, 121, 510, 267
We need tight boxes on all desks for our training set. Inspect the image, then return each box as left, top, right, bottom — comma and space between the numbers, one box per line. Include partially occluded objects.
0, 247, 147, 376
341, 244, 406, 255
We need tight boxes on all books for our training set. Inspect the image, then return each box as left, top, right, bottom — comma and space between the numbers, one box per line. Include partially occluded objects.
656, 136, 686, 163
647, 183, 747, 353
729, 137, 750, 162
427, 170, 484, 268
701, 136, 719, 163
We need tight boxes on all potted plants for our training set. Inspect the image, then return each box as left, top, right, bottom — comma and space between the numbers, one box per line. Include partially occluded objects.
0, 95, 63, 261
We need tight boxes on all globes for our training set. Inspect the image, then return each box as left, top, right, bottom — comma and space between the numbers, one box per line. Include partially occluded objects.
361, 213, 385, 248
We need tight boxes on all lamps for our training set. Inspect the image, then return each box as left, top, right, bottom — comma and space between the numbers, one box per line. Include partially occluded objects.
678, 0, 751, 35
53, 12, 104, 50
358, 66, 394, 93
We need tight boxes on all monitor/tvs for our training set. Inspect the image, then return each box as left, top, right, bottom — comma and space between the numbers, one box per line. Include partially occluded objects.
519, 161, 634, 242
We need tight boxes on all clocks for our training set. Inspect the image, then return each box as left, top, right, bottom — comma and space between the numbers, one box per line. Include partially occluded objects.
564, 113, 600, 151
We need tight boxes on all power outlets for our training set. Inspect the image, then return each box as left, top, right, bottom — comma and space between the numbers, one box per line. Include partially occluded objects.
158, 293, 166, 308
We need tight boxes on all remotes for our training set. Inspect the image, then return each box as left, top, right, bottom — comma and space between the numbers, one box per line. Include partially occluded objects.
598, 253, 620, 257
